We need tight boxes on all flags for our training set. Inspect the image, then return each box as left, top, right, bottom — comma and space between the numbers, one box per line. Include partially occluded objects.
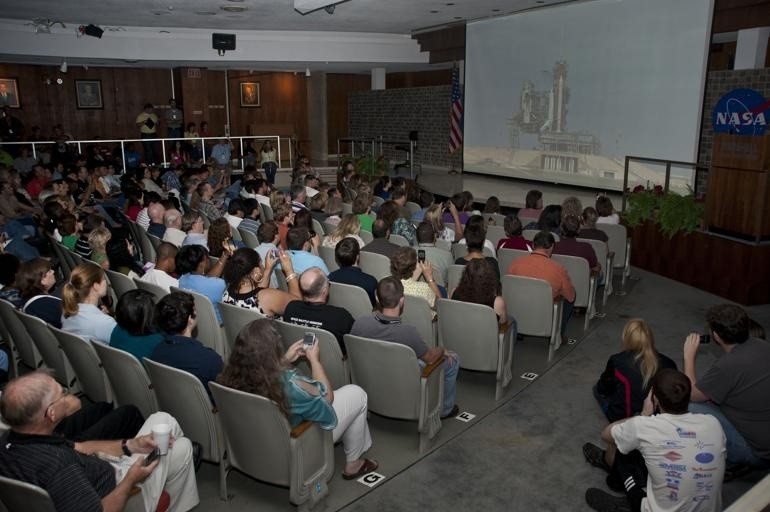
447, 60, 463, 157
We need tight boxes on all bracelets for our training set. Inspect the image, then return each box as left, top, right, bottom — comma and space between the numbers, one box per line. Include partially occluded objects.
285, 273, 296, 282
122, 440, 131, 457
426, 279, 436, 282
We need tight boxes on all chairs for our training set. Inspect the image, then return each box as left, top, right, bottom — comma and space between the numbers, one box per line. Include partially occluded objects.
207, 216, 242, 241
89, 337, 158, 421
482, 214, 506, 227
445, 222, 465, 233
217, 301, 266, 365
274, 268, 300, 293
182, 200, 190, 212
501, 274, 563, 361
144, 231, 161, 252
169, 285, 225, 362
121, 214, 130, 235
317, 245, 340, 273
405, 202, 421, 214
141, 355, 230, 501
236, 227, 259, 249
550, 255, 598, 331
359, 230, 373, 245
388, 234, 410, 247
274, 319, 351, 390
413, 236, 449, 251
132, 277, 169, 304
359, 251, 392, 282
135, 222, 157, 264
522, 230, 560, 243
198, 208, 211, 229
372, 196, 384, 207
447, 265, 466, 291
80, 257, 118, 313
595, 222, 631, 288
519, 217, 538, 227
0, 321, 22, 378
418, 264, 444, 287
260, 202, 273, 222
0, 476, 171, 512
452, 243, 493, 259
575, 238, 616, 306
12, 308, 80, 395
343, 334, 446, 454
65, 249, 82, 266
400, 293, 438, 349
341, 203, 352, 218
208, 380, 335, 512
323, 221, 336, 235
103, 269, 138, 300
326, 281, 373, 321
207, 255, 220, 272
348, 188, 357, 200
497, 248, 528, 282
46, 234, 71, 271
55, 241, 76, 271
47, 322, 112, 404
127, 219, 143, 262
436, 298, 515, 402
486, 225, 504, 245
312, 218, 325, 236
0, 298, 42, 370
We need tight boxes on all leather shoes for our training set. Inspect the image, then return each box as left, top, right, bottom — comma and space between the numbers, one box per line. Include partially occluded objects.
560, 335, 568, 347
441, 406, 462, 421
192, 438, 203, 475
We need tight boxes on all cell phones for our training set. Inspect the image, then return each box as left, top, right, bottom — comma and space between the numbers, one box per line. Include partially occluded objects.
302, 331, 316, 351
143, 447, 160, 466
418, 250, 425, 263
700, 334, 711, 344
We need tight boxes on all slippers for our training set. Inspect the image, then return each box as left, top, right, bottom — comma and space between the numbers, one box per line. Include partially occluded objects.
341, 457, 379, 481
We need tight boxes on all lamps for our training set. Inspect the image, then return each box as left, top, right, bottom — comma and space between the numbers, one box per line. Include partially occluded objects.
56, 78, 63, 86
59, 61, 67, 73
304, 68, 312, 78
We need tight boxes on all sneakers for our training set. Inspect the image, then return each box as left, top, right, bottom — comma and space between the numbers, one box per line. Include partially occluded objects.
583, 441, 614, 474
584, 487, 629, 512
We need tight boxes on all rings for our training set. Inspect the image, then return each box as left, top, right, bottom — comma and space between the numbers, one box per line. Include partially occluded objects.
283, 254, 286, 257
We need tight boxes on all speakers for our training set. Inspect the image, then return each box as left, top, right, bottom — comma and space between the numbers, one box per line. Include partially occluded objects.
212, 33, 236, 50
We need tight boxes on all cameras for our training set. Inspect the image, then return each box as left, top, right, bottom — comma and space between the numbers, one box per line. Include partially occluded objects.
442, 202, 450, 208
271, 249, 282, 259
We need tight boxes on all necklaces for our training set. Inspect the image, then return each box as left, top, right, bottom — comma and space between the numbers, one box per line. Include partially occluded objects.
239, 281, 249, 287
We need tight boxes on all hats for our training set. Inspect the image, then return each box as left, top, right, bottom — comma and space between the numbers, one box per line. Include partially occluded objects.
52, 123, 67, 133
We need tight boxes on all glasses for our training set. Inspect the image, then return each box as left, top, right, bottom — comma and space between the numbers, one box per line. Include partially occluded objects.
42, 386, 71, 419
318, 281, 332, 295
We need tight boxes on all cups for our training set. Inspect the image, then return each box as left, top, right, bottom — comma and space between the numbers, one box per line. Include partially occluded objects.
150, 424, 173, 457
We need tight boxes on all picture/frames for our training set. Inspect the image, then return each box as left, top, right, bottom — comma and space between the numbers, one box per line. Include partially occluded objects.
0, 77, 22, 110
239, 81, 261, 108
74, 79, 104, 110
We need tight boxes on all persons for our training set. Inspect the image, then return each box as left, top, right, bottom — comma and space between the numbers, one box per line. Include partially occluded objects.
168, 141, 186, 166
271, 204, 293, 236
136, 103, 161, 164
593, 318, 679, 423
136, 191, 162, 231
0, 124, 80, 219
224, 165, 270, 206
683, 303, 770, 484
351, 160, 407, 200
238, 198, 261, 237
261, 139, 276, 184
105, 237, 145, 279
175, 241, 234, 323
392, 189, 412, 219
443, 193, 469, 224
60, 262, 118, 345
0, 350, 9, 371
579, 207, 609, 244
293, 209, 322, 257
496, 214, 535, 256
56, 400, 148, 444
207, 216, 244, 258
140, 242, 179, 304
596, 195, 620, 225
165, 99, 184, 150
151, 293, 225, 408
385, 246, 448, 319
144, 161, 225, 202
181, 210, 211, 253
360, 217, 401, 264
351, 277, 459, 421
75, 214, 105, 260
413, 221, 453, 299
275, 226, 331, 279
483, 196, 508, 218
352, 192, 376, 233
80, 134, 144, 228
508, 231, 577, 344
16, 257, 63, 329
451, 257, 517, 346
146, 202, 167, 239
327, 237, 380, 310
216, 317, 380, 481
253, 220, 283, 271
109, 288, 165, 367
283, 266, 355, 358
0, 82, 17, 105
224, 198, 245, 228
198, 121, 212, 160
457, 215, 497, 257
163, 209, 188, 248
239, 148, 257, 173
412, 191, 436, 220
562, 197, 583, 217
211, 139, 234, 170
321, 213, 366, 251
57, 214, 80, 252
464, 190, 482, 217
424, 207, 455, 241
222, 248, 304, 321
88, 226, 112, 269
555, 218, 604, 289
183, 122, 200, 161
377, 200, 415, 244
0, 371, 204, 512
0, 253, 24, 310
523, 205, 566, 239
518, 190, 544, 228
270, 156, 351, 213
583, 368, 727, 512
454, 224, 499, 269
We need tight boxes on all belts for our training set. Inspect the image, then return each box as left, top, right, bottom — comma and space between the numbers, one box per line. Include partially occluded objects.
217, 164, 230, 166
